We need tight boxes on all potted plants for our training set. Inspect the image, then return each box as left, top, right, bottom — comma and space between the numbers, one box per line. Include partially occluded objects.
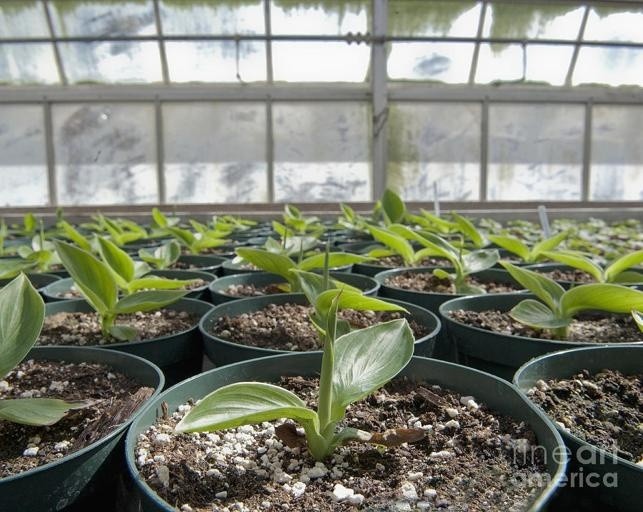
0, 184, 642, 512
